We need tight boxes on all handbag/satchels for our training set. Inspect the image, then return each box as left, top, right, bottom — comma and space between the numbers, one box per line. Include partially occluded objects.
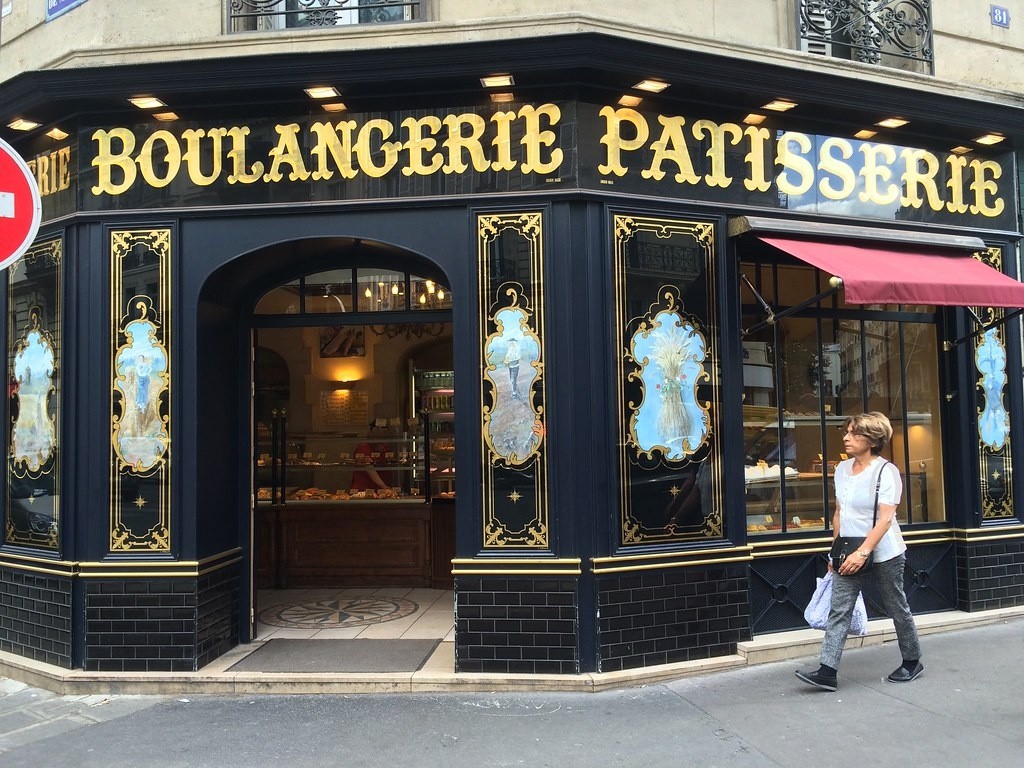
803, 554, 867, 636
830, 537, 875, 573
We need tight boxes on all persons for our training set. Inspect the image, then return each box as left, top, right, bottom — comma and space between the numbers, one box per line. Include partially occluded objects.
794, 413, 926, 690
350, 420, 393, 489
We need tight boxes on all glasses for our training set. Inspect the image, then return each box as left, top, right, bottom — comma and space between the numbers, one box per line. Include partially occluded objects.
841, 430, 866, 438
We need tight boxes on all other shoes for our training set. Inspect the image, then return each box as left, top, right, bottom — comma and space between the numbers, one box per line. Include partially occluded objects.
888, 661, 924, 683
795, 670, 838, 691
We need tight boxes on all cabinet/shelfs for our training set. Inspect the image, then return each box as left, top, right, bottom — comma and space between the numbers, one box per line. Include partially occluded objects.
252, 429, 425, 503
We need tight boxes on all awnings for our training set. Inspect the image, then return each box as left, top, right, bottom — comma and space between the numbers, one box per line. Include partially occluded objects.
755, 236, 1024, 309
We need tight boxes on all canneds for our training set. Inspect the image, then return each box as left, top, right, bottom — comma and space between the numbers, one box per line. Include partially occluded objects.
420, 395, 453, 432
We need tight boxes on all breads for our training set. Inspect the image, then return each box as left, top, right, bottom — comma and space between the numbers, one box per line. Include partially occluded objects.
256, 489, 397, 499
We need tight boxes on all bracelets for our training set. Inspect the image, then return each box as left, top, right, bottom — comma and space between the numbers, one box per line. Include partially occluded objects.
856, 548, 869, 557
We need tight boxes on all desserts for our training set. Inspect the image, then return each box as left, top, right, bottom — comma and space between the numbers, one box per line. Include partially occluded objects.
257, 455, 410, 467
431, 444, 455, 498
